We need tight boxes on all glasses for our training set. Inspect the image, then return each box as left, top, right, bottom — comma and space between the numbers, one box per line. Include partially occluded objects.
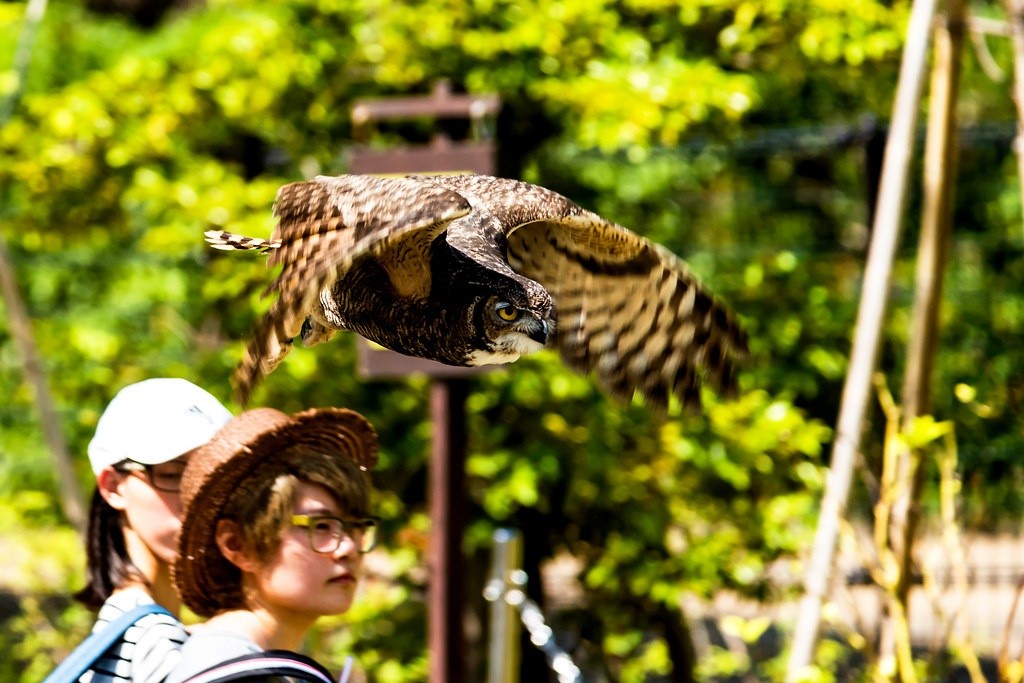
116, 460, 188, 491
292, 513, 376, 554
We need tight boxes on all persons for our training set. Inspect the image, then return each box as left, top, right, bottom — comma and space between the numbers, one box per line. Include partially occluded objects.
156, 405, 381, 683
66, 378, 236, 683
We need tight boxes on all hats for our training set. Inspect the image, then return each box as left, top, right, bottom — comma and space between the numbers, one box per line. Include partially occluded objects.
86, 377, 234, 476
168, 406, 379, 619
